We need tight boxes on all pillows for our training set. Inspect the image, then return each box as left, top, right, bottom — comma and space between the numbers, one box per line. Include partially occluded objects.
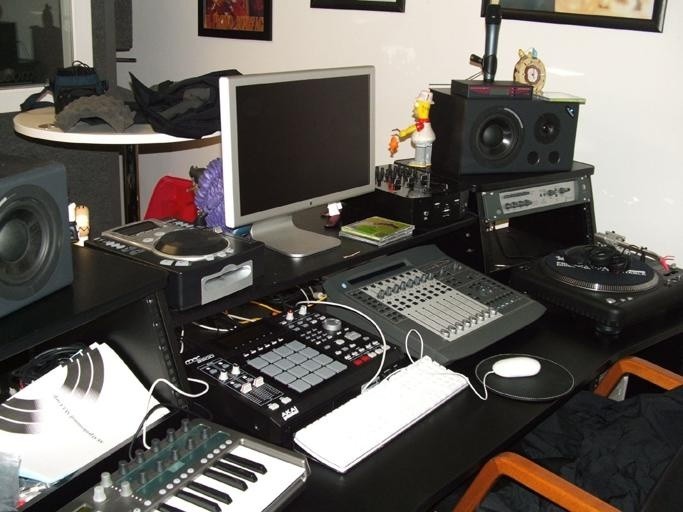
560, 380, 681, 509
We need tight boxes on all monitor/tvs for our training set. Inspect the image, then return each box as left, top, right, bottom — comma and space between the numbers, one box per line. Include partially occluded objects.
217, 64, 377, 261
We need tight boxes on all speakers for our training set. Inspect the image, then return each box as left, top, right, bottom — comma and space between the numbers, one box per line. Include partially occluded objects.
0, 151, 75, 320
428, 87, 580, 182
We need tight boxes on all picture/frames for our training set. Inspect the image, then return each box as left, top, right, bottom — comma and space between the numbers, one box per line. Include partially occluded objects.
197, 1, 272, 41
479, 2, 670, 35
307, 1, 406, 13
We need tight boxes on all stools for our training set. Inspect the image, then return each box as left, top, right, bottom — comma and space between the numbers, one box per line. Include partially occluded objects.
451, 357, 679, 510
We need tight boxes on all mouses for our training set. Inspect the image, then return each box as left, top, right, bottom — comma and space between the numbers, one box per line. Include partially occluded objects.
491, 356, 542, 379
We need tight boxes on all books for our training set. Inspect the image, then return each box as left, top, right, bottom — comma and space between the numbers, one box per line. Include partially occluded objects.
533, 93, 585, 104
338, 229, 411, 247
341, 216, 414, 240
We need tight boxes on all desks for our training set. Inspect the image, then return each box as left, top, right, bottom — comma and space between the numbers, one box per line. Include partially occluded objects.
10, 104, 224, 223
281, 310, 681, 509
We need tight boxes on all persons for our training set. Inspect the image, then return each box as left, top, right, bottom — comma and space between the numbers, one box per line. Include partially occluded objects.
387, 91, 438, 166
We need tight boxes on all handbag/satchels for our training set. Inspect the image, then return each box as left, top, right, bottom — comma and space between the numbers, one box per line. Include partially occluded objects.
21, 61, 103, 111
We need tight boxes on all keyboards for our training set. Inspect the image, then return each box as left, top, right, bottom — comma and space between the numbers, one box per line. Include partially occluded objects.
292, 353, 471, 473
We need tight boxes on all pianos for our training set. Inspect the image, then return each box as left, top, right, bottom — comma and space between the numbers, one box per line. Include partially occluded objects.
18, 418, 313, 511
